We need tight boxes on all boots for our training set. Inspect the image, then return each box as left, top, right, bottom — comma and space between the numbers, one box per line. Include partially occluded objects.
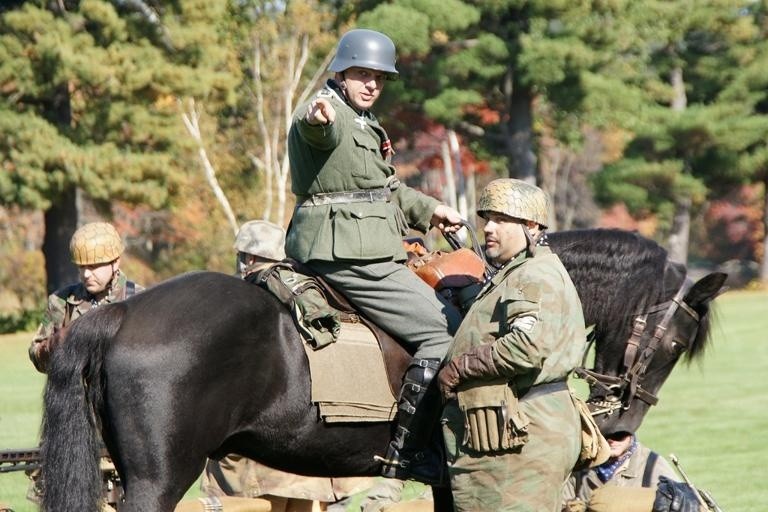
383, 357, 450, 486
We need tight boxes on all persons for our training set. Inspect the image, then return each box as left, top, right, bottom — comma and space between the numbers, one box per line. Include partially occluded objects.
200, 221, 338, 511
565, 426, 716, 511
29, 220, 147, 512
287, 29, 463, 483
437, 178, 589, 512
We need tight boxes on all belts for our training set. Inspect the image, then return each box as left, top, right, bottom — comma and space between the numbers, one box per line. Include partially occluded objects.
295, 188, 394, 205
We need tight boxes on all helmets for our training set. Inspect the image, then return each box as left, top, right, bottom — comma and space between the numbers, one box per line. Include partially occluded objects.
233, 220, 288, 261
68, 222, 125, 267
328, 29, 399, 75
476, 178, 549, 229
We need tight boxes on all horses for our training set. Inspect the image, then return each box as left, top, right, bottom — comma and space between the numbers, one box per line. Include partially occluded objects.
38, 228, 727, 512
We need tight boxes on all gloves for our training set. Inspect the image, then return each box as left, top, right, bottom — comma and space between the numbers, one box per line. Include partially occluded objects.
438, 360, 461, 400
